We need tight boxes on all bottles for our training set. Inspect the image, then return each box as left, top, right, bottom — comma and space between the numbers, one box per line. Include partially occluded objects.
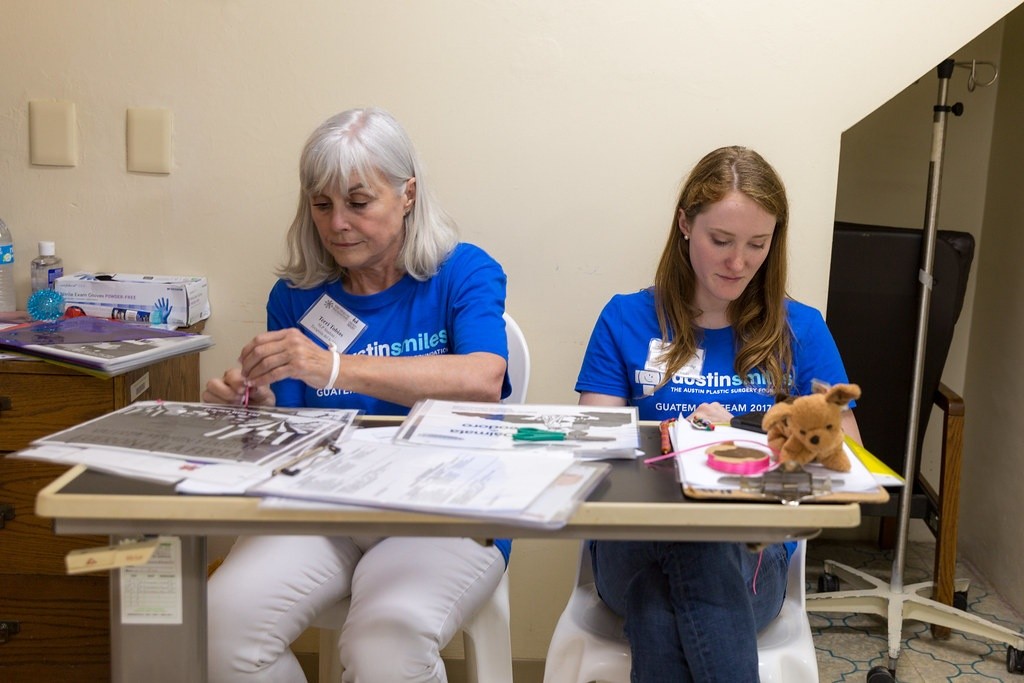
0, 219, 17, 312
31, 241, 63, 294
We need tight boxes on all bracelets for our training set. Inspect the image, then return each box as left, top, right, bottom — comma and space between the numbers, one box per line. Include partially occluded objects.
323, 341, 341, 389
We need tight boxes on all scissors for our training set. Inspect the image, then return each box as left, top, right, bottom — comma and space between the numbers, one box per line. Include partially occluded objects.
512, 427, 617, 443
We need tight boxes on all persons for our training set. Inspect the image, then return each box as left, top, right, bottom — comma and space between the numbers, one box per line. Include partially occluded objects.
201, 110, 514, 683
574, 146, 865, 683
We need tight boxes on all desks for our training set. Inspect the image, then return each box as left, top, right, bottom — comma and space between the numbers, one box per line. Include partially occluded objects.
35, 415, 863, 683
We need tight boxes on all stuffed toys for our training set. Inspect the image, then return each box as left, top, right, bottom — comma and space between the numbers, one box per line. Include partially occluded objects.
762, 382, 862, 472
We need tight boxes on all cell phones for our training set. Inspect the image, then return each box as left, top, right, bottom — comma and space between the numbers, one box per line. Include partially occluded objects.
730, 412, 768, 435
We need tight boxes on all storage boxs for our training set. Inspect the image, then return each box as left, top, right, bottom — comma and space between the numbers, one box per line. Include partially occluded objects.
55, 271, 211, 327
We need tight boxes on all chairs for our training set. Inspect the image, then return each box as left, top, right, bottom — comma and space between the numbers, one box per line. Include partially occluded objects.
543, 541, 820, 683
308, 312, 531, 683
814, 222, 978, 640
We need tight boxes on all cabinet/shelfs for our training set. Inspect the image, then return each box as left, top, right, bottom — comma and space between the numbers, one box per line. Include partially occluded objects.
0, 319, 210, 683
